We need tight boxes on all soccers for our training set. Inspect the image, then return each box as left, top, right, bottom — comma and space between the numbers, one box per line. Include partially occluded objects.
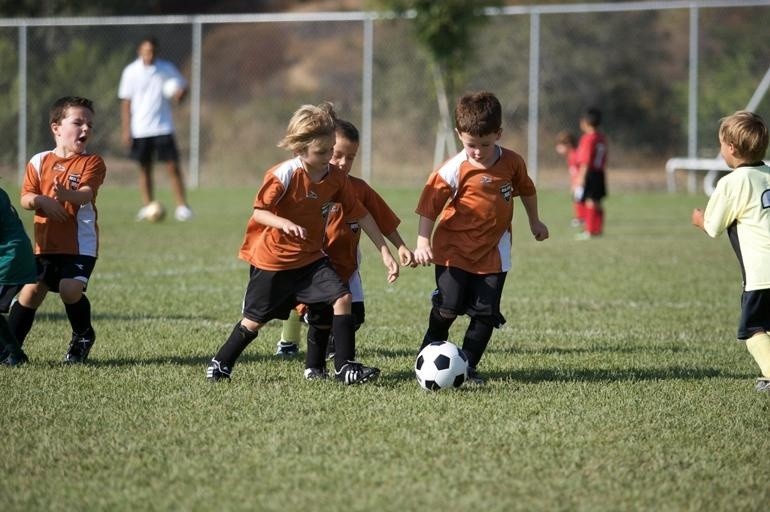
415, 341, 469, 391
161, 78, 181, 98
144, 201, 165, 222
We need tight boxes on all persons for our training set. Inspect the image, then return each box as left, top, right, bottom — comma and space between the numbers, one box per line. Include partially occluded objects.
204, 101, 400, 386
691, 110, 770, 393
0, 97, 107, 363
268, 117, 418, 384
117, 37, 192, 222
411, 89, 547, 382
573, 106, 607, 238
0, 187, 38, 365
553, 127, 584, 230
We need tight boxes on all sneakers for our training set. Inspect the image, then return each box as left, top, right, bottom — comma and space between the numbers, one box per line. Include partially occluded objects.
65, 330, 94, 362
138, 208, 152, 221
466, 364, 479, 378
176, 207, 195, 221
206, 335, 379, 387
754, 376, 770, 391
1, 346, 30, 366
